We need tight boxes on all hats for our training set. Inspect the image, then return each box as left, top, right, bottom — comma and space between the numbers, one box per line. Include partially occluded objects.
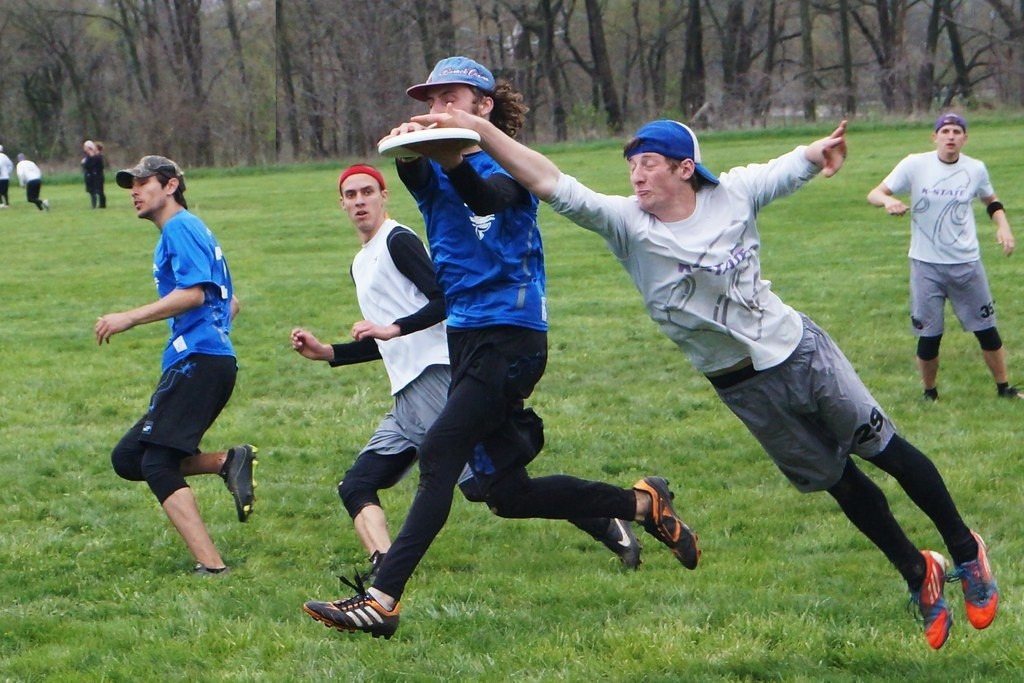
627, 119, 719, 185
406, 57, 495, 101
116, 156, 183, 188
935, 114, 965, 134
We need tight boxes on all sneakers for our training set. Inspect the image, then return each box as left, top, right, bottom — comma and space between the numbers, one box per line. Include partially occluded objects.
193, 560, 231, 576
362, 550, 387, 582
224, 445, 258, 522
594, 518, 643, 571
303, 568, 400, 640
905, 550, 952, 649
944, 530, 999, 629
632, 476, 701, 570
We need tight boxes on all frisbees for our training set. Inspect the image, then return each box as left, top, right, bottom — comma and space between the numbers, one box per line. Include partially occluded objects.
379, 126, 483, 158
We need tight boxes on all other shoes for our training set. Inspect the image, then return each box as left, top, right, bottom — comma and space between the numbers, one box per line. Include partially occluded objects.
43, 200, 49, 209
0, 203, 8, 208
998, 383, 1024, 399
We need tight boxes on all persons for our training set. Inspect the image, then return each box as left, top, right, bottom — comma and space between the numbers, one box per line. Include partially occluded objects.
16, 153, 49, 210
81, 140, 107, 208
301, 57, 705, 640
868, 114, 1024, 404
0, 145, 13, 208
289, 162, 644, 573
410, 104, 1000, 651
95, 155, 262, 577
